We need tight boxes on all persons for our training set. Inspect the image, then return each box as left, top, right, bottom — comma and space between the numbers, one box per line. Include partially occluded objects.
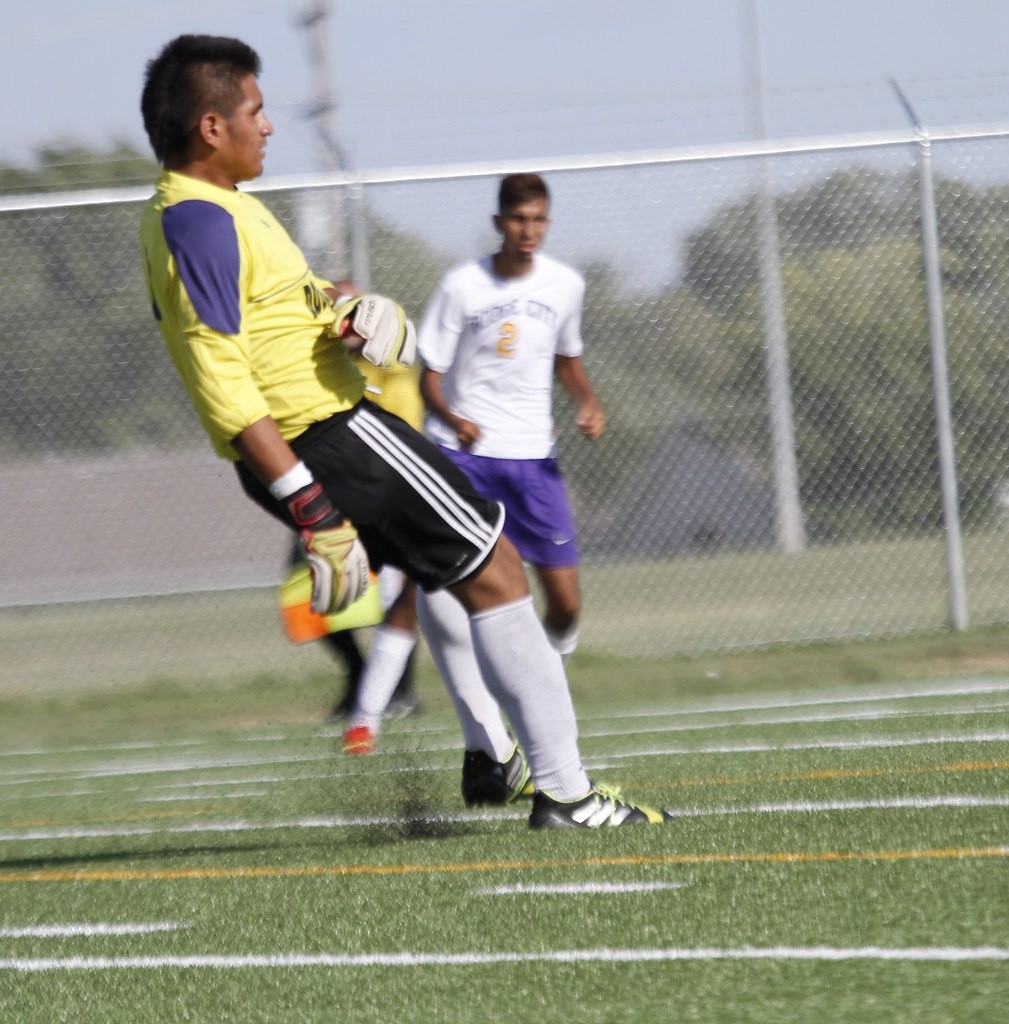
142, 35, 674, 832
291, 279, 420, 755
417, 173, 604, 665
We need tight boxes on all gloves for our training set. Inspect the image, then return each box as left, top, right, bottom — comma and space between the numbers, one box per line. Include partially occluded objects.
327, 294, 423, 364
279, 481, 370, 615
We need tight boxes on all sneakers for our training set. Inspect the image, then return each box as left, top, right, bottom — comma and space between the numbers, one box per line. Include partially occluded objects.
530, 782, 674, 825
463, 745, 537, 807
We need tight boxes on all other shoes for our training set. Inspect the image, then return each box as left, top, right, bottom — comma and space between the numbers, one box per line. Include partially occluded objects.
340, 728, 372, 757
